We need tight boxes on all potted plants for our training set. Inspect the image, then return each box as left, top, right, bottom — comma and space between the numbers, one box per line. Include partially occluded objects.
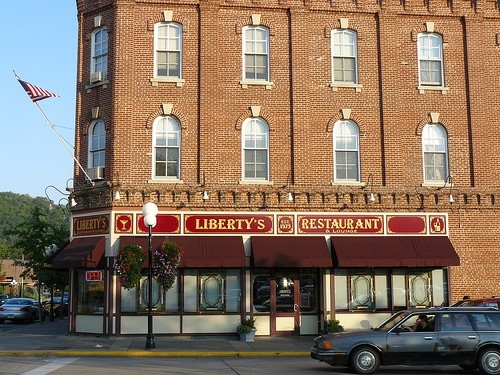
238, 317, 256, 343
326, 318, 345, 336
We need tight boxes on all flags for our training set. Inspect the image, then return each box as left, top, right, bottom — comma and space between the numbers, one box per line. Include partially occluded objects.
15, 74, 60, 103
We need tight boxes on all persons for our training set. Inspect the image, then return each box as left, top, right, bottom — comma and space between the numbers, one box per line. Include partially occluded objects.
403, 314, 434, 332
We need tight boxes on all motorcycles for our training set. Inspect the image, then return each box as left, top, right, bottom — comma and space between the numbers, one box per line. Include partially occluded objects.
42, 300, 64, 319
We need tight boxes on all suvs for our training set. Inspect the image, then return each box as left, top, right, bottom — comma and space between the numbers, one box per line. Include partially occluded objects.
316, 305, 500, 375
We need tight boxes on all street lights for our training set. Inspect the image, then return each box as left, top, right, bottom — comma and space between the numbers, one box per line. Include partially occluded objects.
143, 201, 158, 350
49, 244, 57, 322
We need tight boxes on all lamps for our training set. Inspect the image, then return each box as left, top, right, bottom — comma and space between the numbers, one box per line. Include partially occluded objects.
66, 178, 83, 209
362, 173, 376, 202
285, 172, 294, 202
43, 186, 72, 209
202, 170, 210, 200
114, 171, 121, 200
438, 175, 455, 204
59, 197, 71, 221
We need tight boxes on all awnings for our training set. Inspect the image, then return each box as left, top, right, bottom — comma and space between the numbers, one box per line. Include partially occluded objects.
53, 236, 106, 270
250, 236, 332, 268
330, 236, 460, 267
120, 236, 248, 269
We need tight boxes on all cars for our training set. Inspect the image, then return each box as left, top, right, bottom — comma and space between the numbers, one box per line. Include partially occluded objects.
0, 298, 44, 324
53, 292, 69, 303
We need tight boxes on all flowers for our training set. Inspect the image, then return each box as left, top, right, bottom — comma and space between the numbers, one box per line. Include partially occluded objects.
115, 244, 142, 290
154, 239, 184, 292
